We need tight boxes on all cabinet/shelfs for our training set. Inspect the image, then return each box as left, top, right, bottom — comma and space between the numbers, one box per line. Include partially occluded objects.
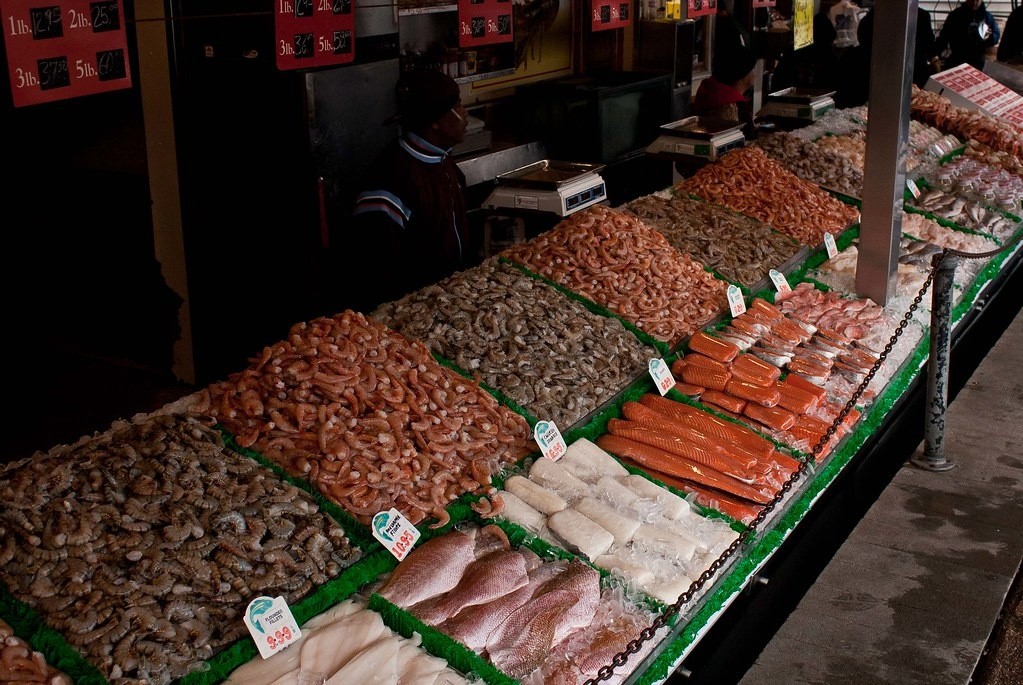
639, 19, 696, 119
399, 5, 516, 86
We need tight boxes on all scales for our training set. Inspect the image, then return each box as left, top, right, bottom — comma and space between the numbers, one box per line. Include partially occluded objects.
755, 87, 837, 122
645, 115, 748, 162
479, 159, 608, 218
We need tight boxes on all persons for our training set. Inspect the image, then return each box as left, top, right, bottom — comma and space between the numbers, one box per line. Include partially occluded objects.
692, 43, 759, 141
996, 0, 1023, 62
936, 0, 1000, 71
857, 4, 935, 88
332, 68, 519, 317
813, 4, 838, 48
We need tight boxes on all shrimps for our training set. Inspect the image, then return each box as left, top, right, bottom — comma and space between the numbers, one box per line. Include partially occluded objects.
0, 130, 865, 685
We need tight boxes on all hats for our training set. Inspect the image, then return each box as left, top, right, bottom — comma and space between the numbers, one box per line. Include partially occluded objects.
395, 69, 460, 129
712, 48, 755, 82
